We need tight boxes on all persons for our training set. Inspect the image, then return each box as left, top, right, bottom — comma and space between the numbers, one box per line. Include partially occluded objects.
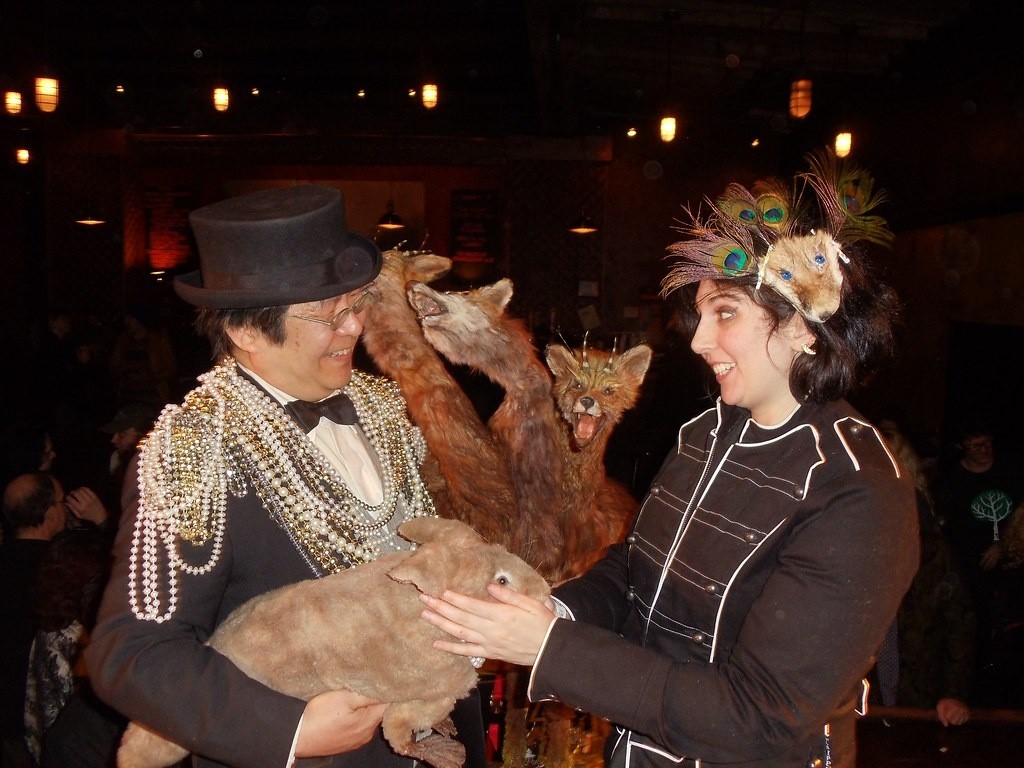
0, 414, 143, 768
860, 418, 1024, 766
420, 181, 922, 768
83, 187, 486, 768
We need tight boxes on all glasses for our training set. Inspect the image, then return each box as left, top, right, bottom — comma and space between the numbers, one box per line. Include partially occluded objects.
275, 286, 373, 332
967, 438, 994, 452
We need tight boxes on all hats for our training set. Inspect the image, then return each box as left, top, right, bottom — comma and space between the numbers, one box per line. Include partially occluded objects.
174, 186, 381, 309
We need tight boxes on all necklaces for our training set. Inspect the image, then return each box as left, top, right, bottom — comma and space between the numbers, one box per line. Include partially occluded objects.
128, 354, 485, 670
